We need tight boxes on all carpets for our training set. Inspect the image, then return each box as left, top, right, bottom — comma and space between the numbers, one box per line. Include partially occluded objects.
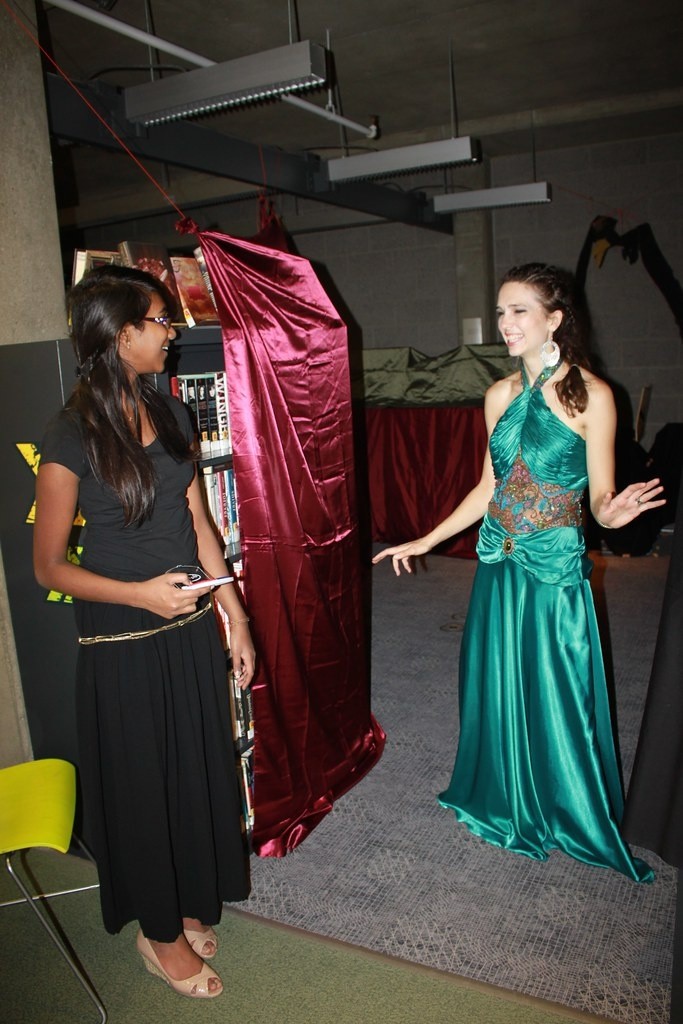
221, 542, 683, 1024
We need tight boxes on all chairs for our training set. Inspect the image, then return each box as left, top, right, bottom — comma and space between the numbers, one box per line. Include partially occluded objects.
634, 383, 651, 442
0, 753, 107, 1024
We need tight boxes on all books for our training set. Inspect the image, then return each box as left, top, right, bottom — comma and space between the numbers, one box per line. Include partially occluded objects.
240, 745, 254, 829
212, 560, 244, 651
229, 665, 254, 740
203, 462, 240, 546
173, 371, 232, 453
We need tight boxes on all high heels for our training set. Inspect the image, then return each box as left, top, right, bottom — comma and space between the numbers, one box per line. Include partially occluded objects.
182, 919, 219, 959
138, 929, 224, 998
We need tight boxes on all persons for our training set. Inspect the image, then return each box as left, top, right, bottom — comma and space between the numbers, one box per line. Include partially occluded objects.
33, 263, 255, 998
372, 263, 666, 884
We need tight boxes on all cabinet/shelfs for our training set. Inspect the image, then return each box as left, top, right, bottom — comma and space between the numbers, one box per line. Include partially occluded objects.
0, 320, 385, 867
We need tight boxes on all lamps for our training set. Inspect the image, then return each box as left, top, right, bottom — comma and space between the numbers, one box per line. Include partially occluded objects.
328, 135, 552, 215
123, 38, 328, 129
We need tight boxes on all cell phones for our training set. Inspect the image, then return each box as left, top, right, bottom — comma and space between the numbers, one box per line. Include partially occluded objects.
166, 565, 235, 590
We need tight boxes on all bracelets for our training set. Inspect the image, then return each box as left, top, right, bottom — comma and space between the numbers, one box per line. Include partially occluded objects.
598, 520, 611, 529
229, 617, 250, 625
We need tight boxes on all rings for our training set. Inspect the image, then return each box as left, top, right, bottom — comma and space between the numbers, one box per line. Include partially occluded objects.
635, 496, 646, 504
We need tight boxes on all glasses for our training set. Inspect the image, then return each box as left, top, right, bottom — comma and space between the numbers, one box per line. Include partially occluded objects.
136, 314, 173, 332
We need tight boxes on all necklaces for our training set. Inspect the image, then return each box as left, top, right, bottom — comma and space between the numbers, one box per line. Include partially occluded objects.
127, 400, 140, 422
